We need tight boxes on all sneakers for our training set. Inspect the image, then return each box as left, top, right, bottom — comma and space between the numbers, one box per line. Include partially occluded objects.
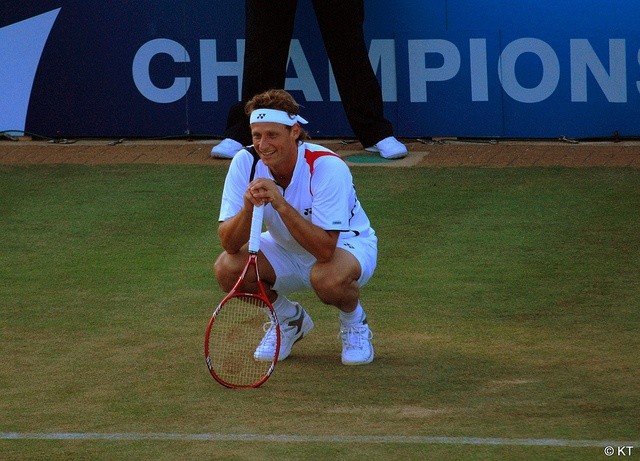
253, 301, 315, 363
208, 137, 244, 158
365, 134, 408, 159
335, 310, 374, 366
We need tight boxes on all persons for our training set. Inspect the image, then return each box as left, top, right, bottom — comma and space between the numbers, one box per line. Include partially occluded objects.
210, 0, 408, 160
213, 89, 378, 367
